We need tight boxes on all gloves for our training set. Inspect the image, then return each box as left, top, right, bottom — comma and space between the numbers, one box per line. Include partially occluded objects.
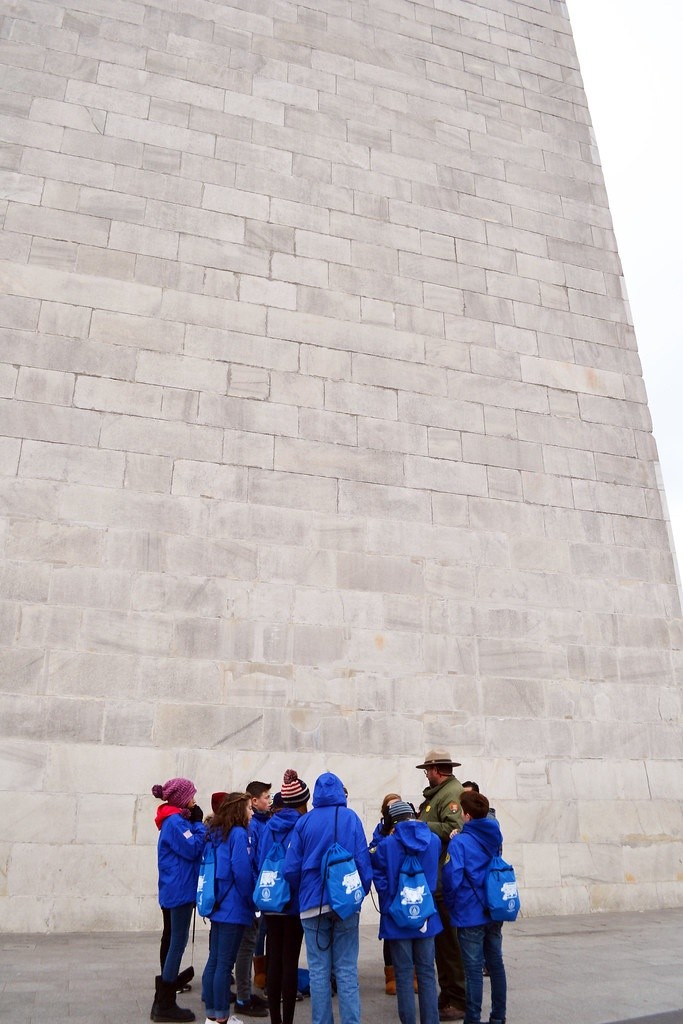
190, 805, 203, 823
380, 806, 398, 837
407, 801, 418, 819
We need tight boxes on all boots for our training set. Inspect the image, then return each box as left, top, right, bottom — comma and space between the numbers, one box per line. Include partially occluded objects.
267, 989, 282, 1024
254, 955, 266, 988
282, 985, 298, 1024
413, 965, 418, 993
384, 965, 396, 995
150, 976, 196, 1022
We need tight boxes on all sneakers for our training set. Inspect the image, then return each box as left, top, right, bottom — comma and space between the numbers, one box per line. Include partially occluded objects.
205, 1017, 216, 1024
215, 1014, 244, 1024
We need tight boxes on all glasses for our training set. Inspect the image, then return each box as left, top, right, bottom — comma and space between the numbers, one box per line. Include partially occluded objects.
424, 766, 434, 774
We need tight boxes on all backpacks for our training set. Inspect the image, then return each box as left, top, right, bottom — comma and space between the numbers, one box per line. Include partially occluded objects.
196, 832, 235, 916
252, 831, 292, 913
389, 845, 437, 929
321, 805, 366, 921
464, 830, 520, 921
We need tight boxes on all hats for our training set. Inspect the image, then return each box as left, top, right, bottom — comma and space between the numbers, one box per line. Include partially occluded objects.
151, 778, 197, 808
389, 801, 414, 817
281, 769, 310, 808
416, 749, 461, 769
211, 792, 228, 813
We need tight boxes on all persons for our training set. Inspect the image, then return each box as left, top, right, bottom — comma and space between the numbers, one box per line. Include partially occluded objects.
152, 749, 508, 1024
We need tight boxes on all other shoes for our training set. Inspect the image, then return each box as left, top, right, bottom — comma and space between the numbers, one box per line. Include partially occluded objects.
234, 994, 268, 1016
295, 990, 304, 1001
438, 997, 465, 1020
